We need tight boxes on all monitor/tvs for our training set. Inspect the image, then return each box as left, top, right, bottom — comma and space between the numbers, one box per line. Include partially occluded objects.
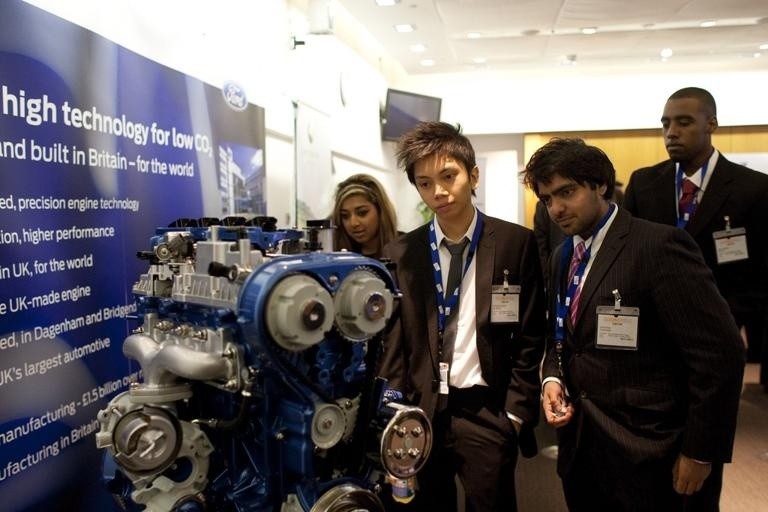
381, 87, 442, 142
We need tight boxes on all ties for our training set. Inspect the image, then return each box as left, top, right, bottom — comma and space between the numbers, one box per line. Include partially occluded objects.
679, 180, 699, 220
566, 242, 585, 327
435, 244, 465, 412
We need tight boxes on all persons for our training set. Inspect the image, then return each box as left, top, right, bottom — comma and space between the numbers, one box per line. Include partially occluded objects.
532, 186, 623, 287
518, 136, 746, 512
331, 174, 409, 257
624, 87, 768, 357
375, 121, 548, 512
